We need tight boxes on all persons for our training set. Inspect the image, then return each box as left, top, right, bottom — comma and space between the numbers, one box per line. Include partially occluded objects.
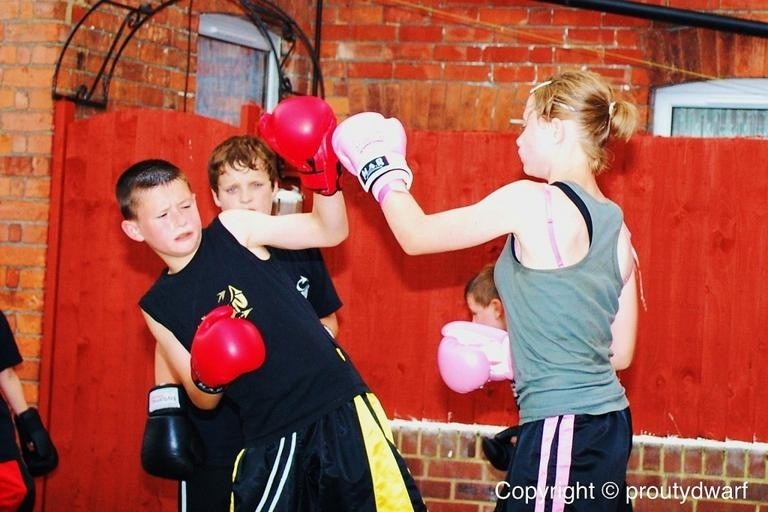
1, 304, 57, 510
110, 92, 434, 511
136, 135, 351, 511
463, 257, 538, 480
327, 69, 651, 512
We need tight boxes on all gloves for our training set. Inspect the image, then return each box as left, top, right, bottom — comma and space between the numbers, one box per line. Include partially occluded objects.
438, 320, 512, 392
13, 405, 60, 476
140, 382, 209, 482
483, 424, 520, 472
190, 304, 268, 392
257, 95, 347, 197
332, 111, 414, 200
